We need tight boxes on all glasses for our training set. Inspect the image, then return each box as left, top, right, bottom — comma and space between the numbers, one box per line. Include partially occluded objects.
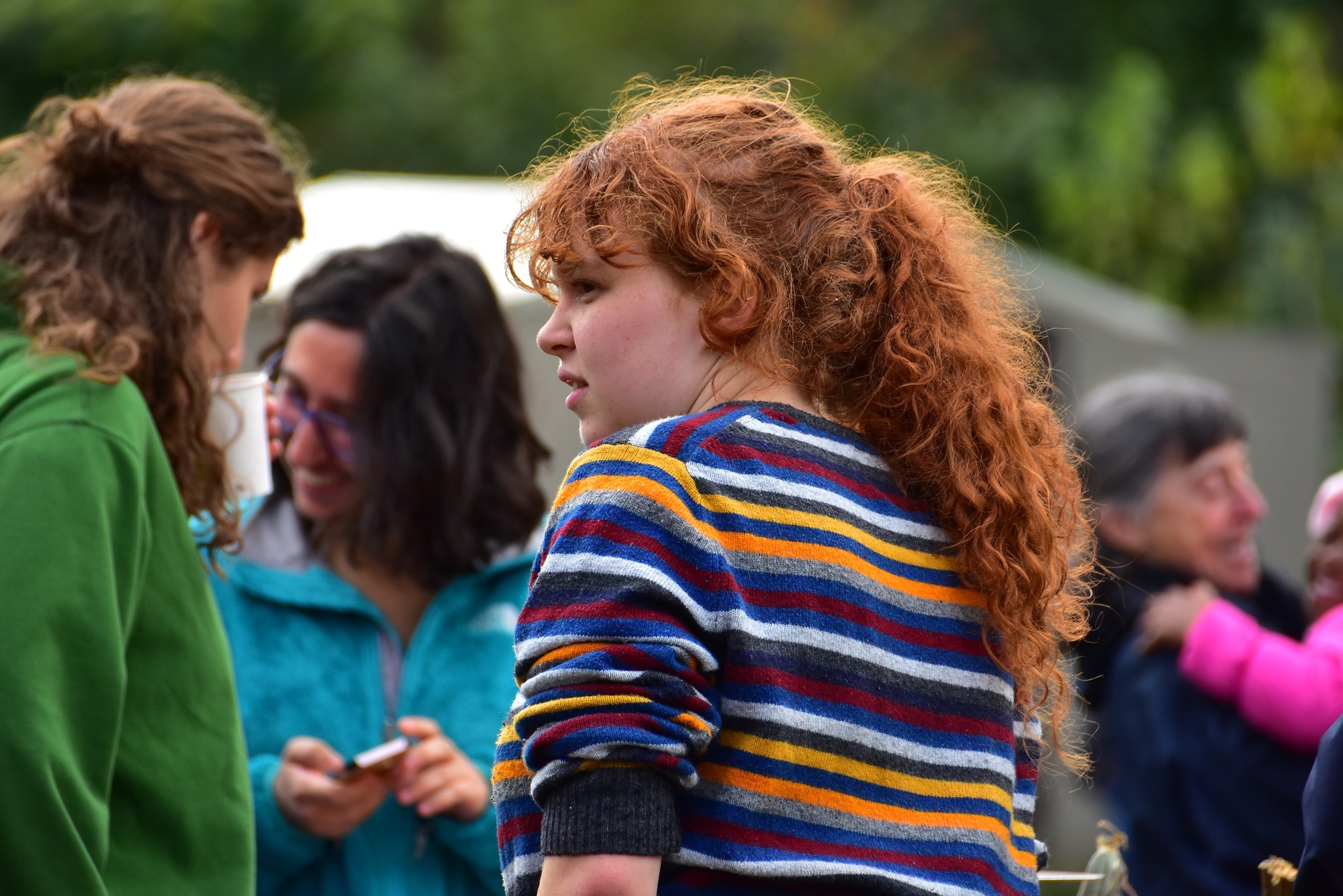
255, 346, 360, 454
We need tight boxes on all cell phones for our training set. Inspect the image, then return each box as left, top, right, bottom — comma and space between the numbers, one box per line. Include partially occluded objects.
329, 736, 409, 778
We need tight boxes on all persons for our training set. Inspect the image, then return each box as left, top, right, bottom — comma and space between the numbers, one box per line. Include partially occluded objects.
0, 71, 304, 896
1042, 365, 1343, 895
189, 234, 556, 896
492, 75, 1092, 896
